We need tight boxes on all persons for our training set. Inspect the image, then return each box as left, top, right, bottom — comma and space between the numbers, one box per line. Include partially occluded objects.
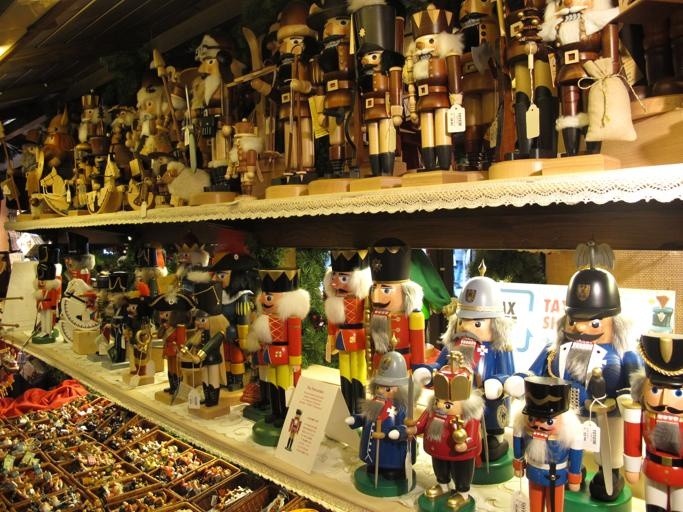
0, 2, 682, 512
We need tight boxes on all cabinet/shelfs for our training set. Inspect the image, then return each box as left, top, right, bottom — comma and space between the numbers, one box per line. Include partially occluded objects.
0, 162, 683, 511
